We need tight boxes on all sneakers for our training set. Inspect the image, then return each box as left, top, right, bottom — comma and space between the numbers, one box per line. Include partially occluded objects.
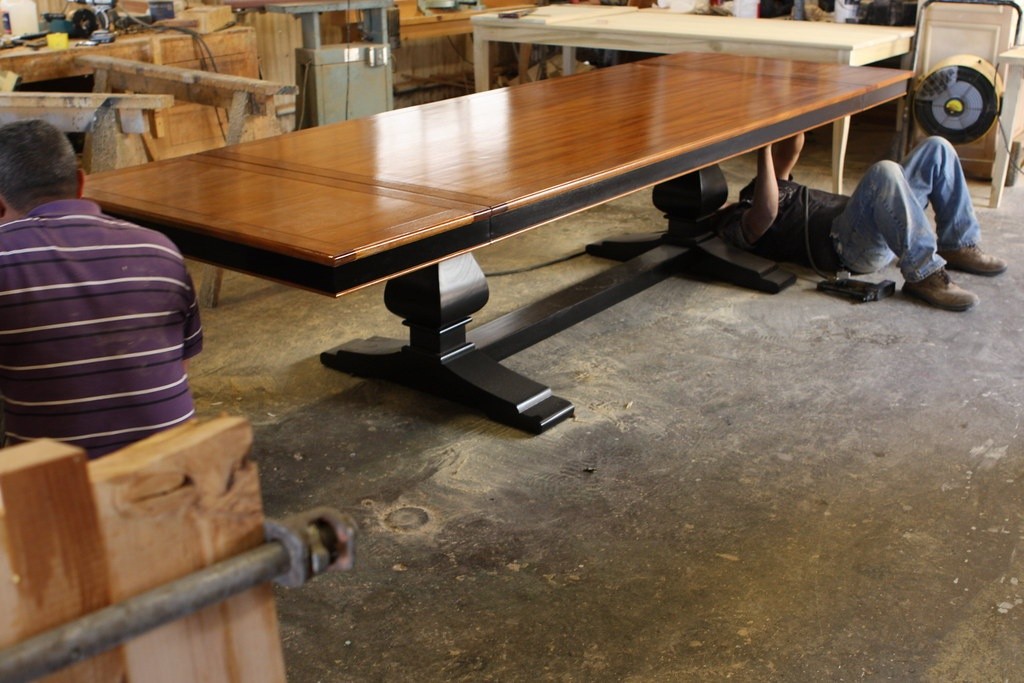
938, 244, 1008, 278
902, 266, 980, 312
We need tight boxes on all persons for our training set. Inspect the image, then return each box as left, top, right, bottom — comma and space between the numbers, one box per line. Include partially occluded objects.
0, 119, 204, 462
70, 0, 96, 38
716, 130, 1008, 311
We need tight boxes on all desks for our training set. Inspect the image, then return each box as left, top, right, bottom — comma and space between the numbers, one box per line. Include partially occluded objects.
79, 50, 915, 437
988, 47, 1024, 208
470, 4, 917, 195
329, 0, 537, 110
260, 0, 395, 132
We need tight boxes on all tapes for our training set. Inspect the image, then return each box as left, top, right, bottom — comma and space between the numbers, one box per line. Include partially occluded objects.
45, 32, 69, 50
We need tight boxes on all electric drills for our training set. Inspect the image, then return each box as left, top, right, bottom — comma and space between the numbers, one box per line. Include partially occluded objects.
815, 276, 897, 302
